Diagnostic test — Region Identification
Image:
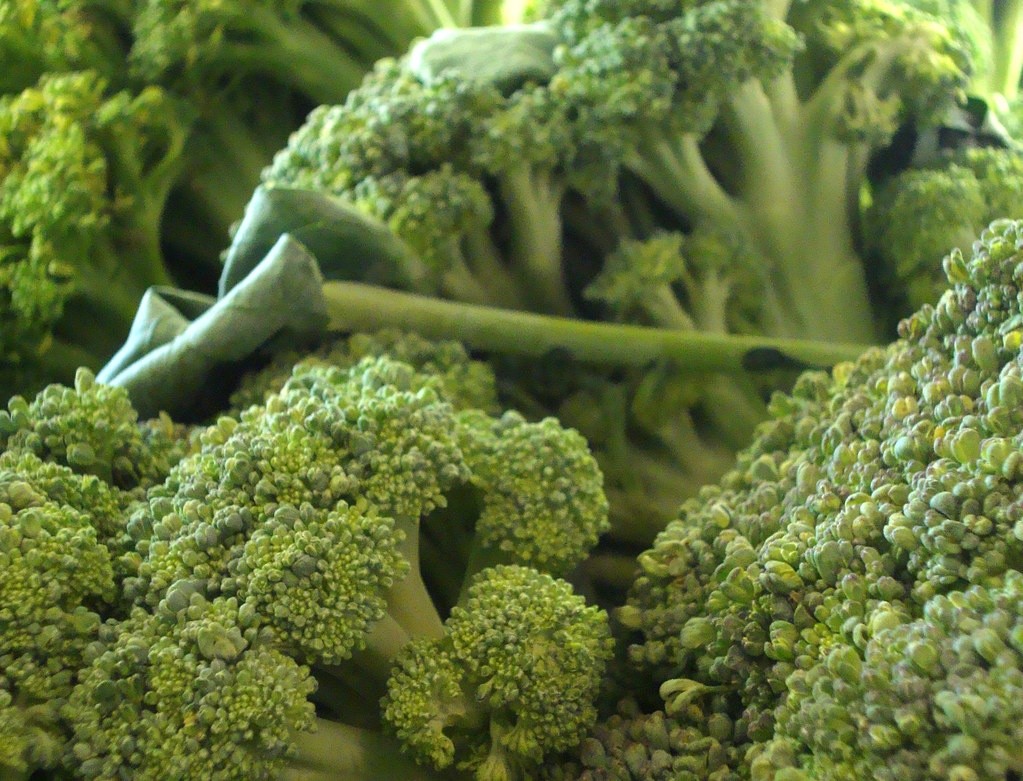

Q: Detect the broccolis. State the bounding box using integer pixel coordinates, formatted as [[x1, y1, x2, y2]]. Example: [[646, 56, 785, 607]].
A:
[[0, 0, 1023, 781]]
